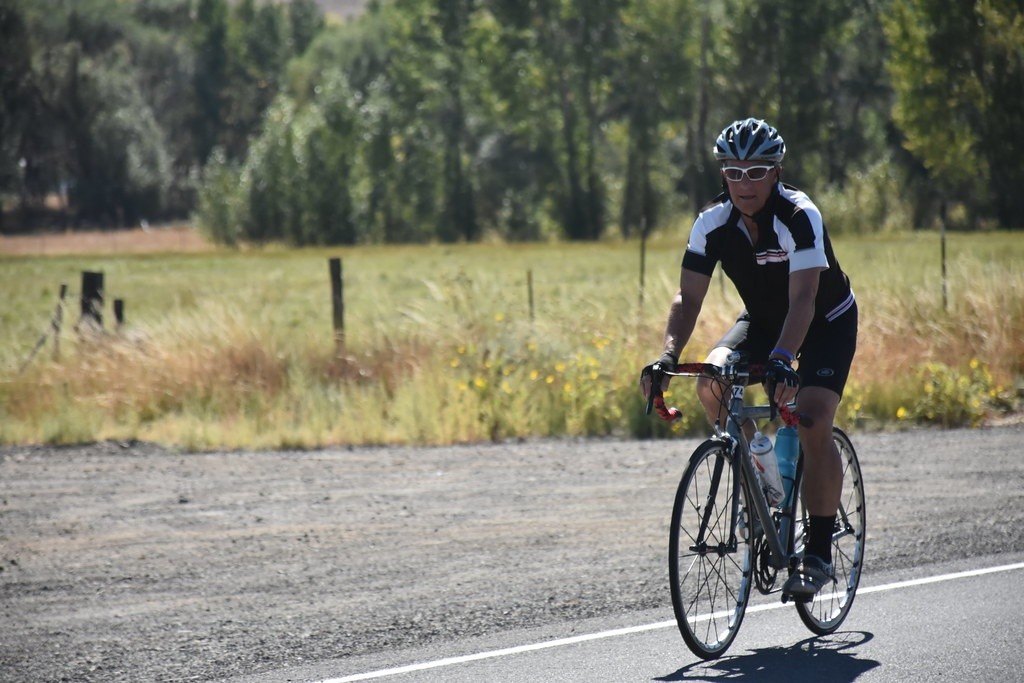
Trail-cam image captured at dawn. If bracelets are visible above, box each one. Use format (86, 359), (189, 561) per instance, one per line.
(771, 349), (794, 359)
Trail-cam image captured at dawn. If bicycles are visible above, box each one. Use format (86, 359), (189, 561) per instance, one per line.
(642, 359), (871, 662)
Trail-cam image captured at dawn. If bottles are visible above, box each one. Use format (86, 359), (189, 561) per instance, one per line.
(773, 426), (799, 508)
(750, 432), (785, 507)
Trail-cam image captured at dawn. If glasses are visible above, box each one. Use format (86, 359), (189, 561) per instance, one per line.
(720, 160), (775, 182)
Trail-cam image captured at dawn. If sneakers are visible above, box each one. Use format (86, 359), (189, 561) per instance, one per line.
(779, 554), (833, 594)
(739, 492), (770, 540)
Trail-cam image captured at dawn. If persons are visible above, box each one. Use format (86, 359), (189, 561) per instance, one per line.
(639, 117), (858, 595)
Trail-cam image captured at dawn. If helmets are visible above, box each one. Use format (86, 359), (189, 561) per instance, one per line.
(713, 118), (787, 162)
